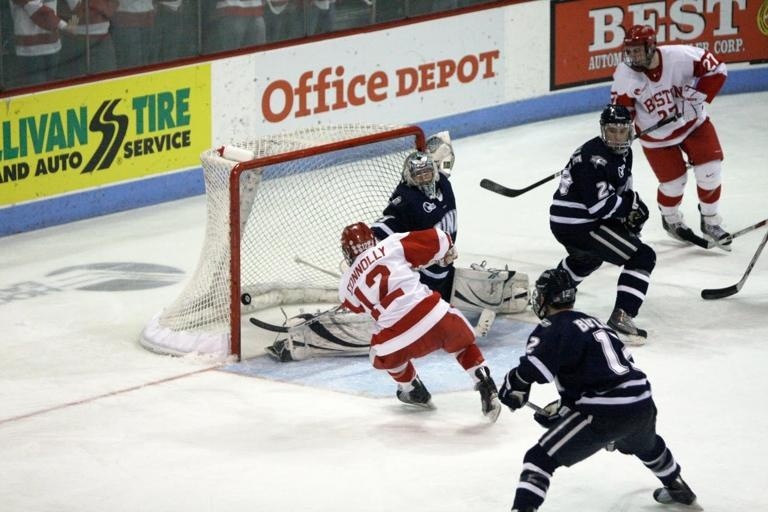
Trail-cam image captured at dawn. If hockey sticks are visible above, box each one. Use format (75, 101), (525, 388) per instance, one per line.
(498, 389), (617, 451)
(479, 110), (683, 198)
(700, 234), (767, 300)
(249, 248), (453, 333)
(672, 218), (767, 250)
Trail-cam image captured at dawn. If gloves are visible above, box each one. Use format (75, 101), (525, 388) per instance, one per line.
(682, 82), (708, 122)
(498, 367), (531, 412)
(626, 200), (650, 235)
(533, 399), (562, 430)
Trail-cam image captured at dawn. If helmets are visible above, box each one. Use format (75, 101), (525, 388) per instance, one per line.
(599, 104), (632, 153)
(532, 269), (577, 318)
(623, 24), (656, 69)
(402, 152), (440, 195)
(340, 221), (377, 268)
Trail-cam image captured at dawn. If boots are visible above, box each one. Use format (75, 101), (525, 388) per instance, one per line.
(658, 206), (695, 244)
(698, 204), (734, 252)
(653, 470), (698, 508)
(605, 308), (649, 347)
(475, 367), (501, 426)
(395, 374), (437, 412)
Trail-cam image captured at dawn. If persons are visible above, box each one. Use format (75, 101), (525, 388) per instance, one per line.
(10, 1), (373, 87)
(338, 223), (499, 416)
(550, 105), (656, 345)
(499, 268), (697, 512)
(265, 150), (531, 364)
(609, 24), (732, 246)
